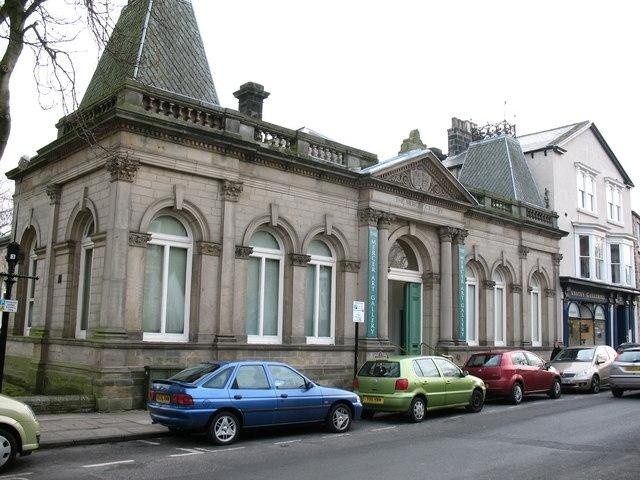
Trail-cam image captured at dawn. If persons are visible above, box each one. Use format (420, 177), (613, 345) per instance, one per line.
(550, 341), (564, 361)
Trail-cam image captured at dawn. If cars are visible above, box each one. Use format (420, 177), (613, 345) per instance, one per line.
(462, 349), (561, 405)
(353, 355), (486, 422)
(549, 344), (618, 393)
(147, 360), (363, 445)
(610, 342), (640, 396)
(0, 393), (43, 472)
